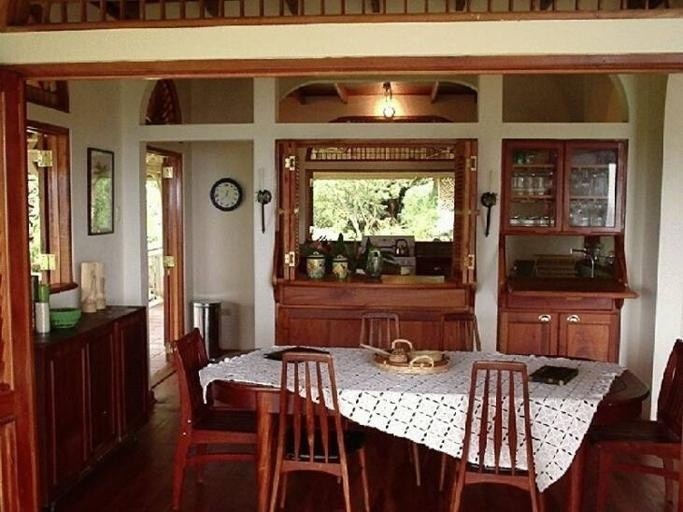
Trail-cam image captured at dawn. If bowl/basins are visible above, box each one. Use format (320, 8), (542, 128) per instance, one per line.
(49, 308), (82, 329)
(508, 216), (549, 226)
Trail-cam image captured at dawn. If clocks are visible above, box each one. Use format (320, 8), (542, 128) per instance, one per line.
(211, 179), (242, 213)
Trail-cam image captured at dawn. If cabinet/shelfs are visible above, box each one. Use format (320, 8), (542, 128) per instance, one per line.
(502, 138), (640, 297)
(498, 293), (621, 364)
(272, 275), (476, 353)
(32, 304), (149, 501)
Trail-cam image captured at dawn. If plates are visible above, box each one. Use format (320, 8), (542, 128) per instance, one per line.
(371, 349), (451, 375)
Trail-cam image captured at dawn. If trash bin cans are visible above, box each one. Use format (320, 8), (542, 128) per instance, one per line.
(189, 299), (222, 360)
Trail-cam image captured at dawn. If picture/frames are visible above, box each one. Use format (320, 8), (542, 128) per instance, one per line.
(86, 147), (116, 235)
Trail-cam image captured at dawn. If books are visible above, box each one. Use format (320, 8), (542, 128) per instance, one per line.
(264, 346), (330, 364)
(529, 365), (579, 387)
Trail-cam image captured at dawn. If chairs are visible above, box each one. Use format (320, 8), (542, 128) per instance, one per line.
(586, 337), (683, 512)
(438, 312), (481, 352)
(440, 361), (541, 511)
(266, 351), (370, 512)
(168, 330), (278, 512)
(336, 312), (422, 486)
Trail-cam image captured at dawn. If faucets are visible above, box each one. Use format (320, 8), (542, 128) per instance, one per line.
(570, 245), (615, 278)
